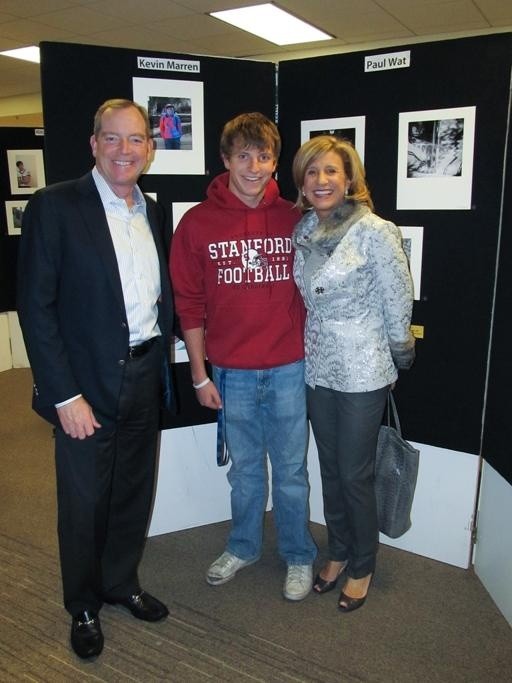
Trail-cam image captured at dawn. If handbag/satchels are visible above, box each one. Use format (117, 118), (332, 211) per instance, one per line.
(373, 425), (420, 539)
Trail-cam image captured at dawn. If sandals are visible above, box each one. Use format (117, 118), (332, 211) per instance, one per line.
(337, 567), (375, 614)
(312, 558), (350, 594)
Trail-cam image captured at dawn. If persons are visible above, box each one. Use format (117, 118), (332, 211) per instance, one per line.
(13, 98), (176, 662)
(168, 111), (321, 601)
(292, 136), (417, 613)
(159, 103), (184, 149)
(16, 160), (31, 189)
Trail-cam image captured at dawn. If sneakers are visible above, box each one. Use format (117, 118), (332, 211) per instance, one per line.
(206, 550), (262, 586)
(283, 565), (313, 601)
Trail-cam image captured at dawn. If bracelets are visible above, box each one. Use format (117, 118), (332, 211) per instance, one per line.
(193, 376), (211, 389)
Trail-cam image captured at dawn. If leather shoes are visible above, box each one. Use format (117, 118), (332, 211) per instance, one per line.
(115, 588), (170, 622)
(71, 613), (105, 662)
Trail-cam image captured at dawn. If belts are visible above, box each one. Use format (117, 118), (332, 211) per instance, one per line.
(128, 335), (166, 360)
